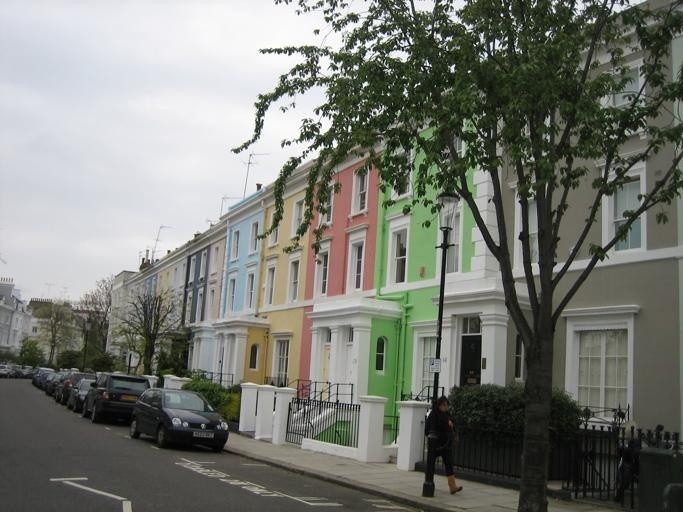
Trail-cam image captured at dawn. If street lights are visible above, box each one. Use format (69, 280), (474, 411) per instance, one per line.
(425, 185), (460, 481)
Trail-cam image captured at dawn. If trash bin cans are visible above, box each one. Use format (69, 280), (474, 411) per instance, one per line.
(639, 447), (683, 512)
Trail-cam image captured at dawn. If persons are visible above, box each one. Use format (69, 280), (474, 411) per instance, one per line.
(424, 395), (463, 495)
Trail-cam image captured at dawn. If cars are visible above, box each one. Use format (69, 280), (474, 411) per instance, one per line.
(0, 363), (151, 422)
(129, 387), (228, 452)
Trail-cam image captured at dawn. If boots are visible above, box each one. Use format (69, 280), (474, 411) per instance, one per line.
(447, 475), (462, 494)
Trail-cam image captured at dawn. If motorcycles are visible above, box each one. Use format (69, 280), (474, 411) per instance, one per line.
(613, 433), (675, 502)
